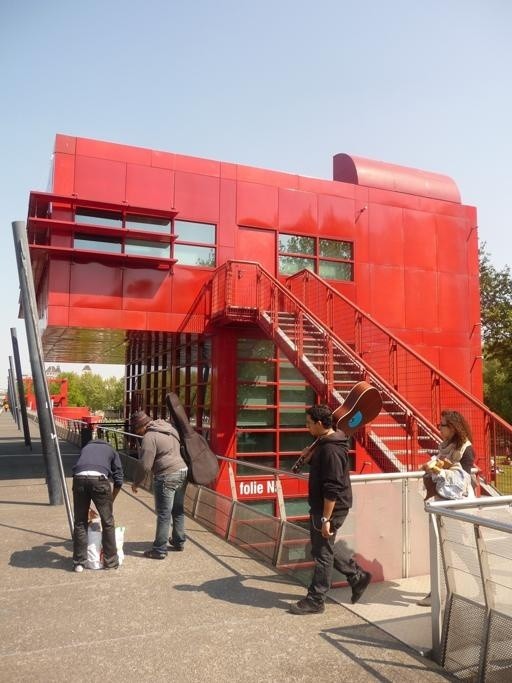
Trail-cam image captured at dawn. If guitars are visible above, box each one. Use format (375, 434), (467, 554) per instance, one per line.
(291, 380), (382, 474)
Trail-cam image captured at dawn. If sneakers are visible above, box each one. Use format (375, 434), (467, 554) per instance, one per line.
(75, 565), (84, 572)
(351, 571), (372, 603)
(418, 591), (433, 606)
(290, 599), (326, 613)
(144, 551), (166, 559)
(169, 536), (184, 551)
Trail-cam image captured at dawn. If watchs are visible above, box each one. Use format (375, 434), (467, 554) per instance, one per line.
(321, 516), (331, 523)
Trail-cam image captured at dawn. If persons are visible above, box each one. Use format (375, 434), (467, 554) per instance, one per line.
(417, 410), (475, 606)
(71, 439), (124, 573)
(129, 411), (190, 559)
(289, 404), (371, 615)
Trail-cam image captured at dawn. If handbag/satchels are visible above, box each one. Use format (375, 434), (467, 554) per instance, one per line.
(422, 473), (436, 500)
(87, 518), (125, 569)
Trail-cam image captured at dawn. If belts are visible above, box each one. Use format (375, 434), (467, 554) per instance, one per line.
(75, 475), (105, 480)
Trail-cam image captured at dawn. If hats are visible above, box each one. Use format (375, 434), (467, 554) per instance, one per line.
(130, 410), (154, 429)
